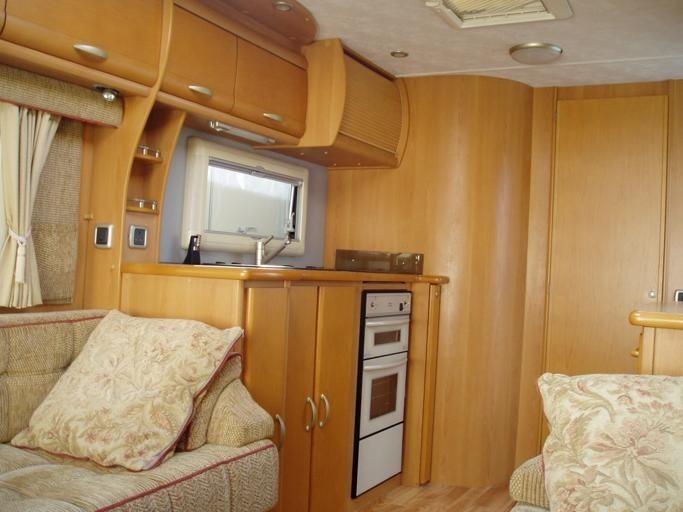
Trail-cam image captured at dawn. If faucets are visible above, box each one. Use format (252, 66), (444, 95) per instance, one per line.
(238, 225), (259, 234)
(262, 236), (292, 264)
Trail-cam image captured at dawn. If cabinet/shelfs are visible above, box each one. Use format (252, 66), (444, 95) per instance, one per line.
(119, 262), (362, 512)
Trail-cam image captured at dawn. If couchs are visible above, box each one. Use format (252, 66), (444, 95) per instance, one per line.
(0, 307), (278, 512)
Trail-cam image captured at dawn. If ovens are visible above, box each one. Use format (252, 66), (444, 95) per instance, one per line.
(352, 289), (414, 498)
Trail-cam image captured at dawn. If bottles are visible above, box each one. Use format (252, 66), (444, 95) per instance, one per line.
(182, 235), (202, 265)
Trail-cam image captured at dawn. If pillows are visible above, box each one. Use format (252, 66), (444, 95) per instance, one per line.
(8, 308), (243, 471)
(534, 367), (683, 511)
